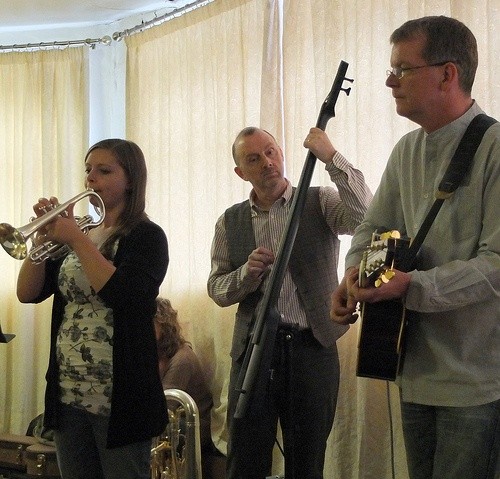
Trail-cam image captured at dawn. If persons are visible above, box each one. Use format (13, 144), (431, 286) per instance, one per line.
(208, 127), (374, 479)
(17, 139), (170, 479)
(330, 15), (500, 479)
(155, 297), (214, 479)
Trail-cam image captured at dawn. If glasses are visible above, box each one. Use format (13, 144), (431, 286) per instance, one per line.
(385, 60), (456, 80)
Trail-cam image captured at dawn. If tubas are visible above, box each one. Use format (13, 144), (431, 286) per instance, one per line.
(150, 388), (202, 479)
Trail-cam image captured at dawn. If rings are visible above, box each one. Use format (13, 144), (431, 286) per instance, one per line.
(307, 138), (311, 142)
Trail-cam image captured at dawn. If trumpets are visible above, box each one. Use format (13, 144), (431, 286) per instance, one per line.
(0, 186), (106, 266)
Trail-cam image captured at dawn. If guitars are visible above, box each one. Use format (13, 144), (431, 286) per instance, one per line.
(354, 226), (411, 383)
